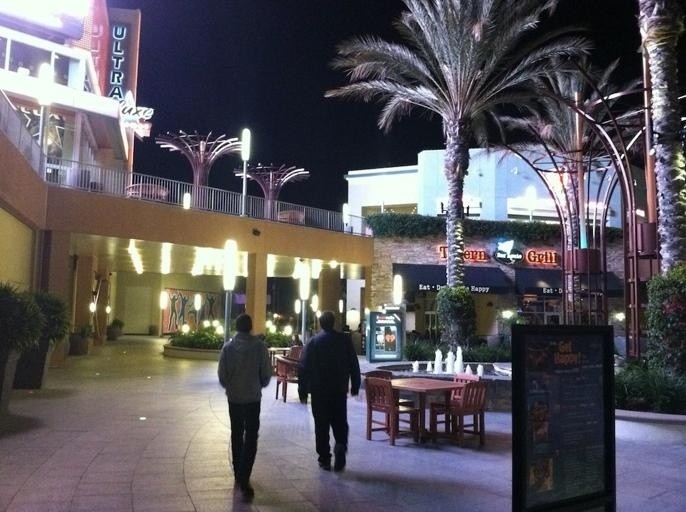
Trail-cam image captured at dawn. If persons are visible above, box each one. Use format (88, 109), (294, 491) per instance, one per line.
(357, 318), (367, 352)
(217, 312), (274, 499)
(295, 311), (362, 473)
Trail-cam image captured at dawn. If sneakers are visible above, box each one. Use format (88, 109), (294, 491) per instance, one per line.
(234, 477), (253, 498)
(333, 461), (346, 471)
(318, 457), (332, 472)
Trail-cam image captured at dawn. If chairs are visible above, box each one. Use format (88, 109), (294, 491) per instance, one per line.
(275, 346), (303, 402)
(364, 377), (420, 446)
(431, 383), (488, 448)
(431, 375), (481, 437)
(365, 371), (416, 434)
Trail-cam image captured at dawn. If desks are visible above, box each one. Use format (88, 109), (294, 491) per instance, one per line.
(387, 378), (467, 444)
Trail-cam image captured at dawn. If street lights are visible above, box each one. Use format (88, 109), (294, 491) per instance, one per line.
(393, 274), (402, 307)
(299, 263), (311, 346)
(221, 238), (238, 345)
(239, 129), (252, 218)
